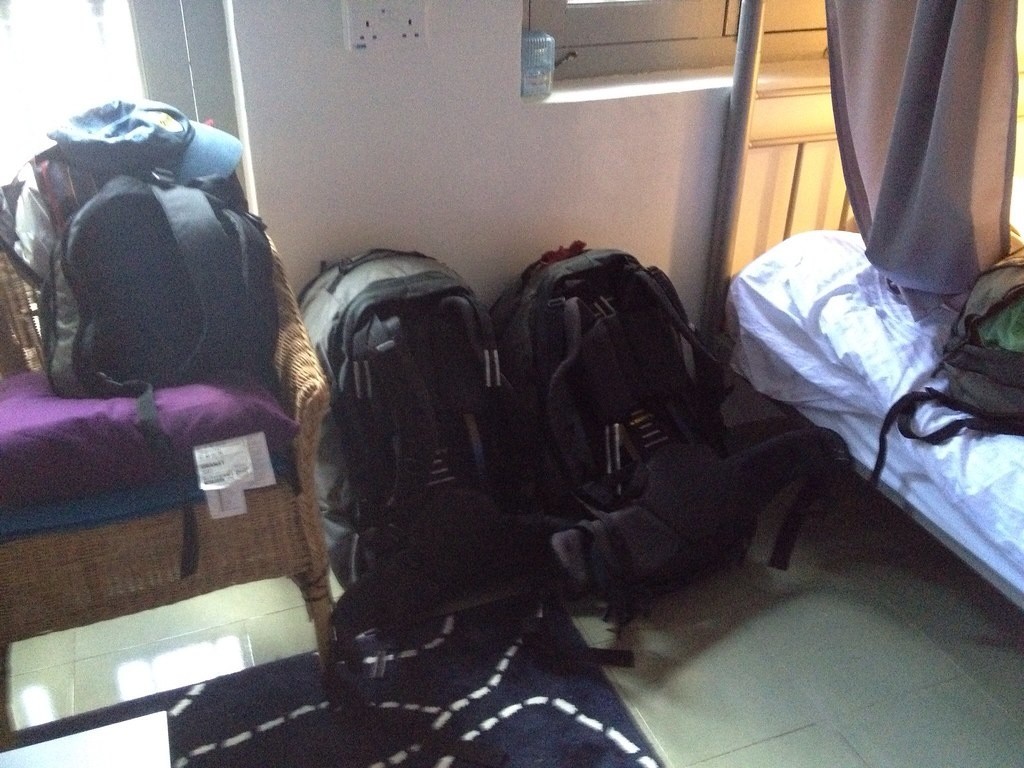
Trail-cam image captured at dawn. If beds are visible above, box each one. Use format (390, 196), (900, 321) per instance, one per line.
(719, 231), (1024, 610)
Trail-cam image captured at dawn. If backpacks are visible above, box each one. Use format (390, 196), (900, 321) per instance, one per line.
(496, 240), (787, 620)
(859, 251), (1024, 507)
(0, 102), (279, 400)
(296, 248), (524, 635)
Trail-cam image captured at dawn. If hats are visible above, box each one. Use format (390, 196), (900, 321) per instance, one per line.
(45, 98), (244, 188)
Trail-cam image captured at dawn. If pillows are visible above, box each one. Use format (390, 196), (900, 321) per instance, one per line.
(1, 379), (303, 494)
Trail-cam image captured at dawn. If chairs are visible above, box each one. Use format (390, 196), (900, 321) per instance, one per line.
(2, 181), (337, 741)
